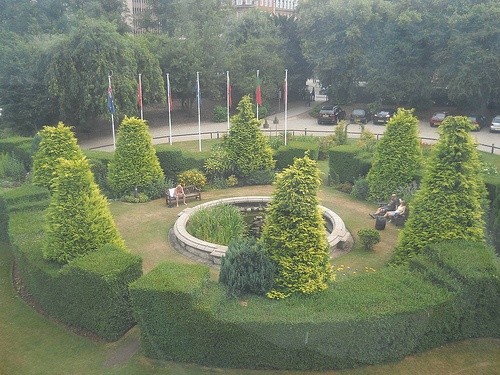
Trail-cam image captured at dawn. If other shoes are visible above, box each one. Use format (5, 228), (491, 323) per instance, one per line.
(184, 203), (187, 205)
(369, 214), (375, 219)
(177, 205), (179, 207)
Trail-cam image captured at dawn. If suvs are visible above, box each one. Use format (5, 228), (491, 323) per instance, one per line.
(317, 105), (346, 125)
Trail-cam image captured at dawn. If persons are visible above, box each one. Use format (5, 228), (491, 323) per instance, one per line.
(369, 194), (400, 219)
(173, 185), (187, 207)
(385, 200), (406, 218)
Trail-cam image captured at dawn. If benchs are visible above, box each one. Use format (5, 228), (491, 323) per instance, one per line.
(165, 186), (201, 207)
(379, 202), (408, 227)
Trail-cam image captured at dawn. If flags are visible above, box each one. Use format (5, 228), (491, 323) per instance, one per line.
(256, 78), (262, 106)
(138, 79), (142, 106)
(107, 81), (115, 116)
(228, 81), (232, 108)
(195, 79), (202, 105)
(284, 78), (287, 97)
(166, 78), (172, 112)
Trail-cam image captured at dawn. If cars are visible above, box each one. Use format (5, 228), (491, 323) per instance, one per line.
(429, 111), (456, 127)
(373, 108), (398, 125)
(319, 88), (326, 95)
(349, 108), (372, 124)
(489, 114), (500, 133)
(465, 115), (487, 131)
